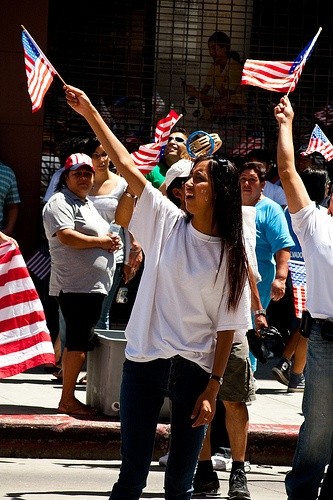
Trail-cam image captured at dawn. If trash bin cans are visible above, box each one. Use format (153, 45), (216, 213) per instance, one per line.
(87, 329), (129, 419)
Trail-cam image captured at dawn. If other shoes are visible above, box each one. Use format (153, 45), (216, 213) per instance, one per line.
(246, 326), (284, 363)
(159, 451), (170, 467)
(272, 356), (291, 386)
(209, 454), (234, 470)
(287, 373), (306, 391)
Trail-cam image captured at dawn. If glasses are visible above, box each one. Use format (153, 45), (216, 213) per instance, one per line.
(168, 137), (186, 143)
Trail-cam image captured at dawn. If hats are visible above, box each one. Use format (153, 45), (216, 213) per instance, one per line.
(65, 153), (95, 172)
(165, 159), (194, 190)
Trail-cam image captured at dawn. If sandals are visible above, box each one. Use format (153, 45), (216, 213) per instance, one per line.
(57, 400), (98, 416)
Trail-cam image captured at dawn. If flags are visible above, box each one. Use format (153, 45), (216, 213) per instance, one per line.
(0, 242), (56, 382)
(115, 109), (183, 176)
(99, 98), (114, 126)
(227, 136), (265, 156)
(26, 245), (52, 280)
(312, 104), (333, 126)
(238, 29), (321, 93)
(300, 124), (333, 161)
(140, 90), (164, 115)
(21, 29), (58, 113)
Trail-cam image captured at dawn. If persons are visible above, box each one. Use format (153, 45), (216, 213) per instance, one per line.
(0, 231), (19, 246)
(270, 94), (333, 500)
(61, 82), (253, 500)
(37, 82), (333, 500)
(0, 161), (22, 236)
(181, 33), (251, 123)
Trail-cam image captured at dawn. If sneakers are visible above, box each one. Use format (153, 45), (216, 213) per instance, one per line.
(228, 464), (252, 499)
(193, 472), (221, 495)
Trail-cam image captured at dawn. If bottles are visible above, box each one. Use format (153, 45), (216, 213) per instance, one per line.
(108, 218), (122, 239)
